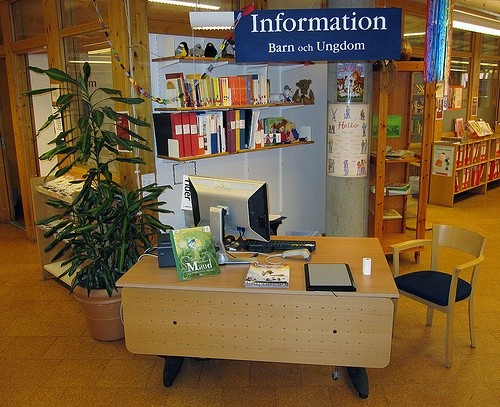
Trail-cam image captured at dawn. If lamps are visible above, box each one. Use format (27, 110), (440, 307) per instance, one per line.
(189, 10), (234, 30)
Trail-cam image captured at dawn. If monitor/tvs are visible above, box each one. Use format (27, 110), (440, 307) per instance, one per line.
(187, 176), (270, 266)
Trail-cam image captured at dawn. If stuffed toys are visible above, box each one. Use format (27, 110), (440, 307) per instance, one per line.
(270, 79), (315, 144)
(176, 32), (236, 57)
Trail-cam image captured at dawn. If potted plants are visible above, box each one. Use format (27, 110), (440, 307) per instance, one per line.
(20, 59), (176, 340)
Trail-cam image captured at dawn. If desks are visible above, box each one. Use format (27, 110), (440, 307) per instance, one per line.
(114, 235), (400, 398)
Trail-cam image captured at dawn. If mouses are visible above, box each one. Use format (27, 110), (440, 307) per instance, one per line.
(225, 235), (235, 245)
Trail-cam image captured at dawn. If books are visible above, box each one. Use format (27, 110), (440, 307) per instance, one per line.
(244, 262), (290, 288)
(170, 226), (220, 281)
(153, 73), (288, 157)
(369, 118), (500, 218)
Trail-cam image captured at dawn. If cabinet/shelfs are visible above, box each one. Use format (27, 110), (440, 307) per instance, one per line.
(408, 68), (500, 209)
(151, 55), (316, 161)
(29, 175), (111, 288)
(369, 60), (435, 263)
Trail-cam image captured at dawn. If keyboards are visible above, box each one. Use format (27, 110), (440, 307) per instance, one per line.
(244, 239), (315, 251)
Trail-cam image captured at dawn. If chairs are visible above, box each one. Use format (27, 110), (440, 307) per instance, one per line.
(387, 222), (490, 369)
